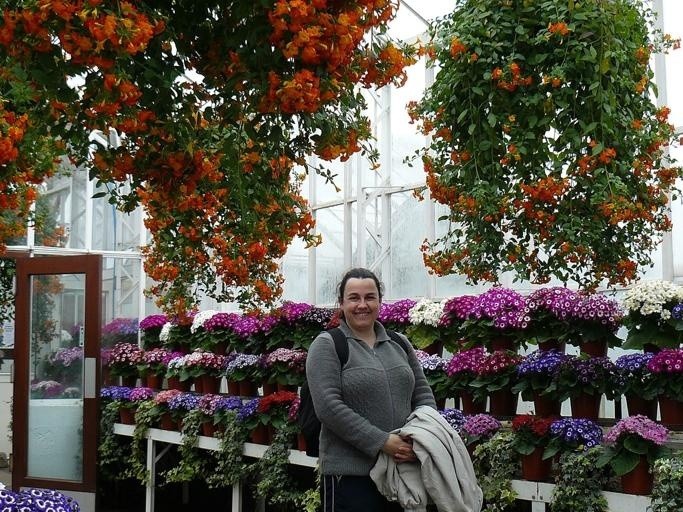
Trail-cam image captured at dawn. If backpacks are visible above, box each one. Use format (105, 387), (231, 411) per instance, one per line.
(296, 327), (409, 458)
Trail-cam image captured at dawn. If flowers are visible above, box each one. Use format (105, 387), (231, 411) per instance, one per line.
(31, 321), (84, 399)
(96, 279), (683, 512)
(0, 488), (80, 512)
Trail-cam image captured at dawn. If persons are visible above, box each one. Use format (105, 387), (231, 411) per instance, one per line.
(305, 268), (437, 512)
